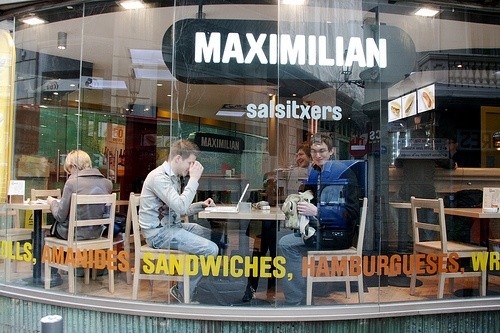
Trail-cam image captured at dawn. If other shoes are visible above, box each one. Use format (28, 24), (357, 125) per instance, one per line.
(41, 272), (64, 287)
(170, 285), (200, 305)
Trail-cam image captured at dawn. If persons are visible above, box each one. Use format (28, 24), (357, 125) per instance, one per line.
(396, 128), (438, 275)
(242, 172), (280, 302)
(442, 139), (464, 168)
(279, 133), (359, 307)
(46, 150), (113, 288)
(296, 141), (313, 192)
(139, 139), (218, 304)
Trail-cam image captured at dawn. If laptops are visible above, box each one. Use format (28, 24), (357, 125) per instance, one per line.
(205, 184), (249, 212)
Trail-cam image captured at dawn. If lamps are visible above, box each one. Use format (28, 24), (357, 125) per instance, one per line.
(58, 32), (67, 49)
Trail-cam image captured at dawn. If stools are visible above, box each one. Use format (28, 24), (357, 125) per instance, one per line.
(390, 201), (420, 256)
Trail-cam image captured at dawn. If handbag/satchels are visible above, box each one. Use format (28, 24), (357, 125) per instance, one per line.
(281, 189), (314, 230)
(114, 211), (133, 235)
(195, 272), (249, 306)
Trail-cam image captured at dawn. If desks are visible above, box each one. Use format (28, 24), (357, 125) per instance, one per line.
(5, 200), (129, 288)
(432, 208), (500, 297)
(198, 204), (285, 306)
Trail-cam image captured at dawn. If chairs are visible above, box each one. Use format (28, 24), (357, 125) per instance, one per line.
(0, 189), (190, 304)
(409, 196), (487, 300)
(306, 198), (368, 306)
